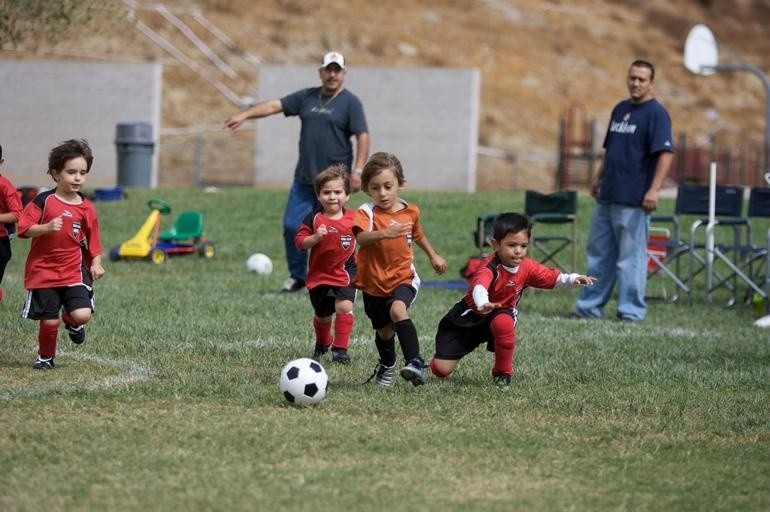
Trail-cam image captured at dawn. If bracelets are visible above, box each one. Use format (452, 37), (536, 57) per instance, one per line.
(353, 168), (363, 174)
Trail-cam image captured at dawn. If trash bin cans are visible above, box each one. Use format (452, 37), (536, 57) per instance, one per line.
(115, 122), (154, 187)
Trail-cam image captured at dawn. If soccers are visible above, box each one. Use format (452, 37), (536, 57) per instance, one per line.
(247, 254), (274, 274)
(281, 359), (329, 405)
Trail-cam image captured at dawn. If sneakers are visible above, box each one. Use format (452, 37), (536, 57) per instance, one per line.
(492, 373), (513, 394)
(374, 355), (399, 389)
(33, 355), (58, 371)
(399, 357), (427, 387)
(314, 340), (334, 362)
(331, 349), (351, 365)
(281, 276), (305, 294)
(61, 306), (85, 345)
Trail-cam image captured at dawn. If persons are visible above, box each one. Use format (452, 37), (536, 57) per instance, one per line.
(568, 60), (675, 321)
(294, 164), (357, 363)
(428, 212), (598, 391)
(16, 138), (105, 369)
(351, 151), (448, 387)
(222, 52), (370, 293)
(0, 145), (23, 301)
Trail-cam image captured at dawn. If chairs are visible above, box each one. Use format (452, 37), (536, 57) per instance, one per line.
(472, 190), (577, 273)
(688, 185), (770, 307)
(646, 186), (744, 306)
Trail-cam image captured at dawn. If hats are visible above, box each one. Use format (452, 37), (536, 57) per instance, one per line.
(320, 50), (346, 72)
